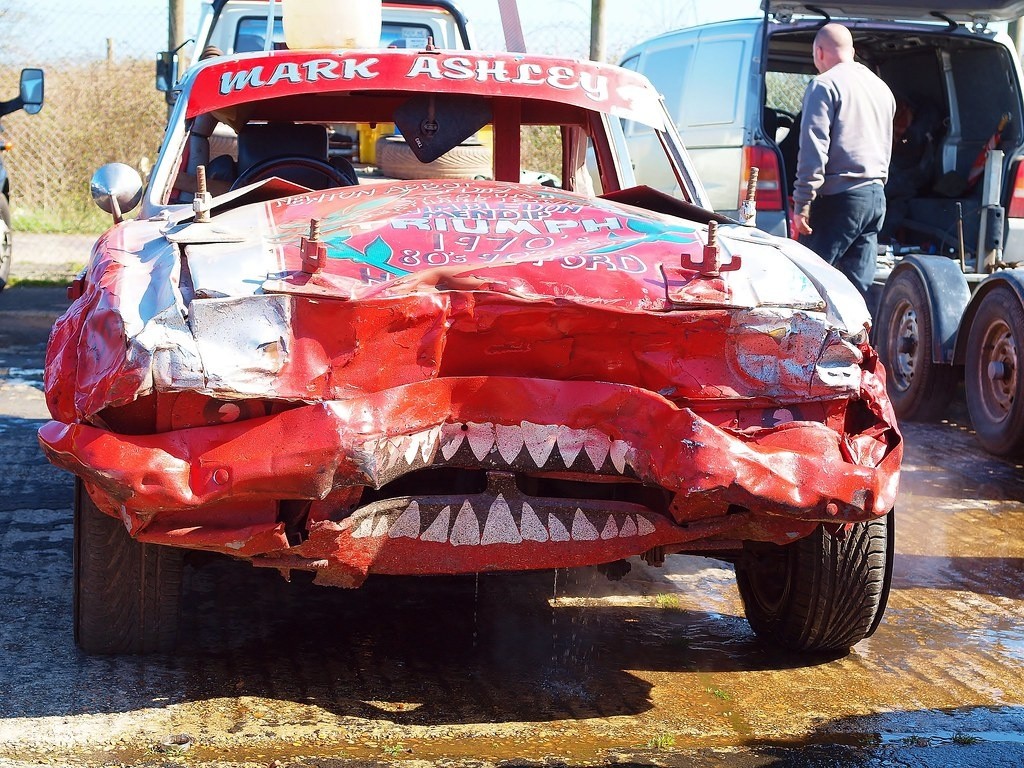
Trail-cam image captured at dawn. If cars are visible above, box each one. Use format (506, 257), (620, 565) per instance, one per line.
(39, 46), (902, 667)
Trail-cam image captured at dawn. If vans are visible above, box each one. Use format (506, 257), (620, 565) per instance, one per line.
(586, 0), (1023, 301)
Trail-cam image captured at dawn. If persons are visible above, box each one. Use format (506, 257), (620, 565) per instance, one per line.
(793, 23), (896, 292)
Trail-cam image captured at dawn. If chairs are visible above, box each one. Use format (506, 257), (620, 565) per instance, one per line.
(237, 123), (329, 199)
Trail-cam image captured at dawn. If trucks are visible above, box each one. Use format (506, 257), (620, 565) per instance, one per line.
(1, 67), (47, 287)
(157, 0), (561, 207)
